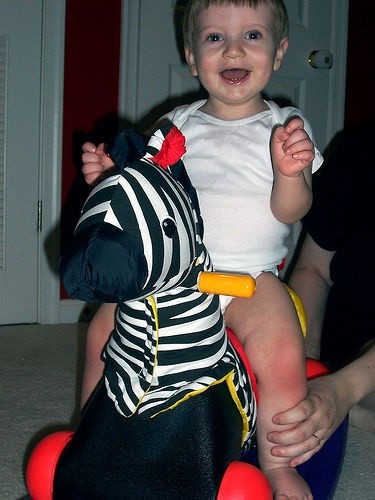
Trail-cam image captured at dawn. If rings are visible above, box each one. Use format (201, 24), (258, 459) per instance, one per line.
(313, 434), (321, 442)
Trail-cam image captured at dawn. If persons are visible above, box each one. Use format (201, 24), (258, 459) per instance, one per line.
(267, 124), (375, 469)
(78, 0), (324, 500)
